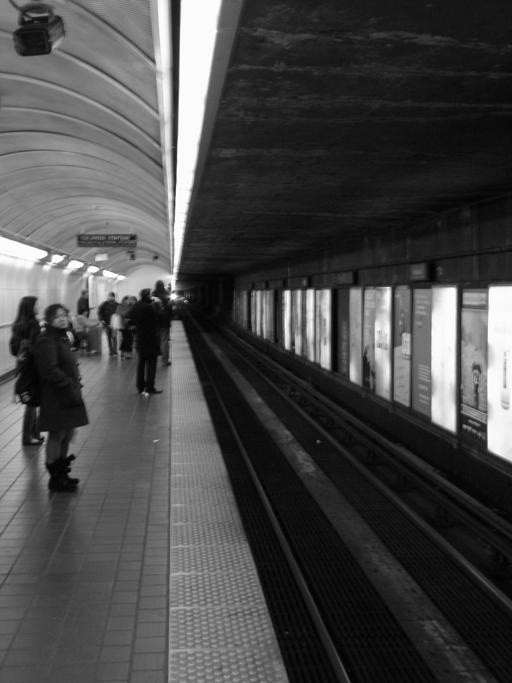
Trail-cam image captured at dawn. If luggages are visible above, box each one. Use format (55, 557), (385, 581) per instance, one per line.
(88, 326), (102, 354)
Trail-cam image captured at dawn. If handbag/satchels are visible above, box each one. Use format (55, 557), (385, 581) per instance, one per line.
(111, 314), (121, 329)
(17, 339), (32, 361)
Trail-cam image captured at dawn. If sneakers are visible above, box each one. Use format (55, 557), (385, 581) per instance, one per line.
(22, 438), (44, 445)
(145, 389), (161, 394)
(138, 388), (144, 394)
(163, 362), (171, 367)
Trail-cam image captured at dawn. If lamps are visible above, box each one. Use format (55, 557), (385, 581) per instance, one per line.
(6, 0), (66, 57)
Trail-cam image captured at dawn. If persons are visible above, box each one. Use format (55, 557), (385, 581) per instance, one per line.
(70, 280), (171, 394)
(12, 295), (45, 446)
(33, 303), (89, 493)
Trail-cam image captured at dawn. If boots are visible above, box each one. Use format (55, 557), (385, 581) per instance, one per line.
(47, 453), (78, 490)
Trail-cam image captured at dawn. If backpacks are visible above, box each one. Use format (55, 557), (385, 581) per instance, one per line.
(15, 357), (39, 406)
